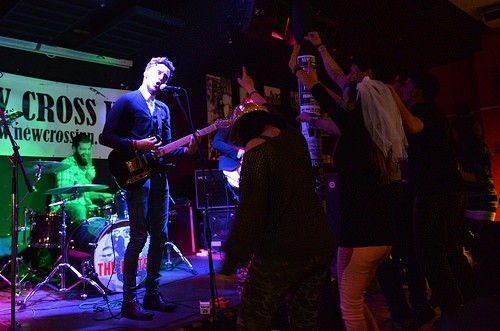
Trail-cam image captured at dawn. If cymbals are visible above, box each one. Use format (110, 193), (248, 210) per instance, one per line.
(44, 185), (108, 195)
(23, 161), (70, 174)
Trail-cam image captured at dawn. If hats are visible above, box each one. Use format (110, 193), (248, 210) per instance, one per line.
(230, 97), (270, 147)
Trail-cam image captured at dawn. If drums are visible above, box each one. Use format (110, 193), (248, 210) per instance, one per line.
(29, 211), (70, 248)
(71, 216), (150, 293)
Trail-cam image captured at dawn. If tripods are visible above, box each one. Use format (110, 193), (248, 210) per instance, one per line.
(19, 183), (107, 311)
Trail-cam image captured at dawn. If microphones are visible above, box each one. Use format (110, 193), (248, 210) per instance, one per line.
(160, 83), (183, 92)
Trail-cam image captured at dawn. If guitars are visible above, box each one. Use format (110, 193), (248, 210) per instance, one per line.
(108, 118), (231, 191)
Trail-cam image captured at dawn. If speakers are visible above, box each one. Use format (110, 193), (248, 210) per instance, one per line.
(194, 169), (240, 209)
(167, 200), (196, 257)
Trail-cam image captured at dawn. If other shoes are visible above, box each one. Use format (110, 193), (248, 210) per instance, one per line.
(392, 319), (415, 331)
(122, 300), (155, 319)
(142, 291), (177, 311)
(411, 307), (434, 327)
